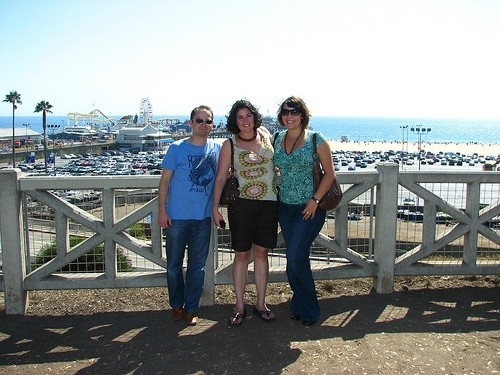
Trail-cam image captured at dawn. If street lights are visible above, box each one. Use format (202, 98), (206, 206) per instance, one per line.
(22, 123), (30, 158)
(47, 123), (61, 177)
(411, 127), (431, 205)
(399, 124), (409, 170)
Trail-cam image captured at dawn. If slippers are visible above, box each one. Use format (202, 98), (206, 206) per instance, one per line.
(228, 308), (247, 327)
(253, 305), (276, 321)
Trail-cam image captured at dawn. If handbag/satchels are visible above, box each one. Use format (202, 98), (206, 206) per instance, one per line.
(311, 132), (343, 212)
(219, 175), (240, 205)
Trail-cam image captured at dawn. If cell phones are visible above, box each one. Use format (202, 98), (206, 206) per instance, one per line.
(220, 220), (226, 229)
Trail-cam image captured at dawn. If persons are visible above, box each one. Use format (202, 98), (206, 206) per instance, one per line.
(271, 96), (335, 325)
(213, 99), (279, 327)
(158, 105), (221, 326)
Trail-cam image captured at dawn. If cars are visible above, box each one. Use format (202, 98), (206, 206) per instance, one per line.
(331, 148), (500, 171)
(2, 135), (168, 205)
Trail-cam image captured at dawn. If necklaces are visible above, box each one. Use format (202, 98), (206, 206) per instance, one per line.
(238, 129), (257, 142)
(284, 128), (304, 156)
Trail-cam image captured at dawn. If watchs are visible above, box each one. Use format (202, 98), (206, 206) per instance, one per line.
(311, 196), (319, 204)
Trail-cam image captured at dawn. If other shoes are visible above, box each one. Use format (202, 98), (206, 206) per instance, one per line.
(173, 308), (184, 321)
(183, 311), (199, 326)
(291, 314), (301, 320)
(302, 320), (315, 326)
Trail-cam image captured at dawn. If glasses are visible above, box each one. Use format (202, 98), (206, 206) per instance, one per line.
(280, 108), (301, 116)
(195, 118), (213, 124)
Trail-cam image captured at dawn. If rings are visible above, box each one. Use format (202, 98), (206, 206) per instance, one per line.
(306, 211), (309, 214)
